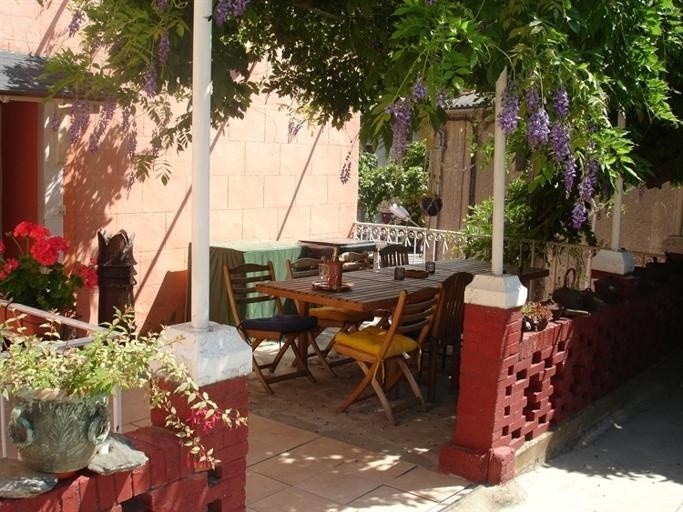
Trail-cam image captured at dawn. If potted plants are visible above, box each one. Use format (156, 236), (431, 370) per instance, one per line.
(521, 302), (553, 332)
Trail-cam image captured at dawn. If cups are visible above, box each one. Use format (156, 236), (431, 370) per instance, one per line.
(425, 262), (436, 274)
(392, 266), (405, 280)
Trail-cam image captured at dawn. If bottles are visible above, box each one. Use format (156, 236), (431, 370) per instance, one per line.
(328, 246), (342, 287)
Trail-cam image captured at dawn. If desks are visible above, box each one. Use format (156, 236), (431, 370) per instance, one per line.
(187, 241), (375, 326)
(257, 257), (550, 397)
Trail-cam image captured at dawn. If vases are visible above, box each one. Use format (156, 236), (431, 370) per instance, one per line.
(0, 300), (76, 341)
(3, 388), (110, 481)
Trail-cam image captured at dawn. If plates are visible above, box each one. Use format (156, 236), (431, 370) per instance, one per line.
(311, 280), (357, 292)
(403, 269), (427, 279)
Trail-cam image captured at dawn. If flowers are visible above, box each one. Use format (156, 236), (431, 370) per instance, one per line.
(0, 293), (250, 470)
(0, 219), (98, 316)
